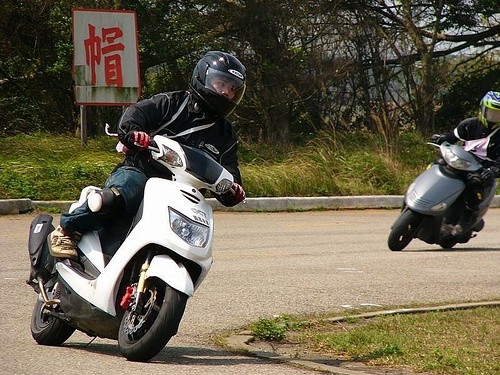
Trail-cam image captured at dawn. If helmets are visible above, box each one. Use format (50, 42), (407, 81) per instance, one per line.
(477, 90), (500, 130)
(189, 50), (247, 118)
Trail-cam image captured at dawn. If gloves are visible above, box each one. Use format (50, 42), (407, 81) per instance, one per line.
(230, 183), (246, 202)
(480, 166), (500, 183)
(432, 133), (451, 145)
(122, 131), (152, 151)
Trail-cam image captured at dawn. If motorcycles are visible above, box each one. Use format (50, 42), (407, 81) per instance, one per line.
(388, 133), (499, 251)
(26, 134), (247, 361)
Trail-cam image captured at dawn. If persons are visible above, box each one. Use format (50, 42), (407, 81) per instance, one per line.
(47, 51), (247, 261)
(432, 91), (500, 238)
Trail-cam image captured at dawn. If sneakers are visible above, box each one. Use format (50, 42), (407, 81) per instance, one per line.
(47, 224), (82, 260)
